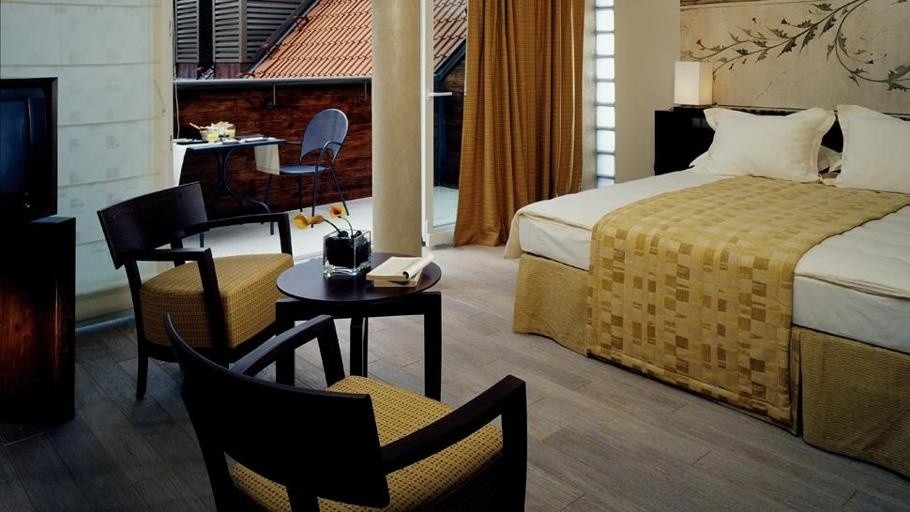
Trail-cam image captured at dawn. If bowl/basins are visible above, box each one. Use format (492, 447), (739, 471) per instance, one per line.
(199, 124), (237, 143)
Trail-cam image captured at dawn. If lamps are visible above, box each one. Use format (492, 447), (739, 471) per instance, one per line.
(674, 61), (713, 107)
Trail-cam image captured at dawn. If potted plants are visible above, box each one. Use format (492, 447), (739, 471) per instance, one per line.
(292, 203), (374, 280)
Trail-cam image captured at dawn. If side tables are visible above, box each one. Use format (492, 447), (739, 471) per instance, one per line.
(275, 252), (442, 402)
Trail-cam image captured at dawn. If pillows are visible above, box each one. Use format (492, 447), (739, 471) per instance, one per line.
(688, 103), (909, 195)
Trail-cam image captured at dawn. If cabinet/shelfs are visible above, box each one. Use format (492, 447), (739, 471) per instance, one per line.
(0, 218), (78, 429)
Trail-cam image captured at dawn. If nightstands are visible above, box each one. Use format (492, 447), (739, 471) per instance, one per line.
(654, 110), (714, 172)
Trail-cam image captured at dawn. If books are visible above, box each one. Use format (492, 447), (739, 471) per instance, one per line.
(365, 253), (435, 289)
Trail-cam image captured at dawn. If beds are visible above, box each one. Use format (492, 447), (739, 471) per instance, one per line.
(503, 106), (910, 480)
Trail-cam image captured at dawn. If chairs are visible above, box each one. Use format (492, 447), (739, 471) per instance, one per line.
(96, 180), (294, 401)
(162, 310), (527, 511)
(261, 108), (349, 228)
